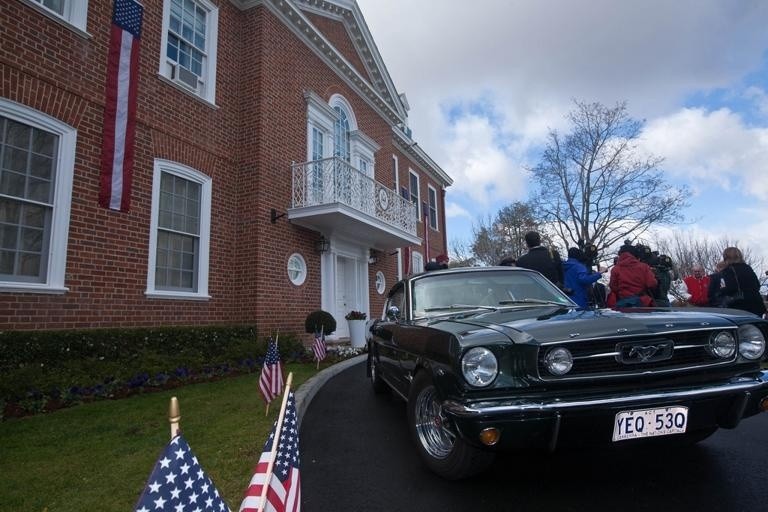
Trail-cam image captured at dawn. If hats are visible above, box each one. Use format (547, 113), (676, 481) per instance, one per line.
(436, 255), (449, 264)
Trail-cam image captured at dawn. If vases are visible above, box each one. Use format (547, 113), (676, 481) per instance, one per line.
(347, 320), (366, 348)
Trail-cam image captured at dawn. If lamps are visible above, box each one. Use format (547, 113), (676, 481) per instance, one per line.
(316, 236), (330, 255)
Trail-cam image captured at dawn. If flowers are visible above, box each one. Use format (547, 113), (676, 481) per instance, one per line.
(345, 310), (367, 320)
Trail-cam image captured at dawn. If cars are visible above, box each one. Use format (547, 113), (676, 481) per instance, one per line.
(365, 266), (766, 482)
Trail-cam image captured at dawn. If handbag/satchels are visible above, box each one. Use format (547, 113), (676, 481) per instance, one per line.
(718, 267), (745, 311)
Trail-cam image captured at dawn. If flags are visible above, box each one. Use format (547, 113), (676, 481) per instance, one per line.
(312, 328), (327, 362)
(258, 336), (285, 405)
(238, 390), (302, 512)
(136, 431), (234, 512)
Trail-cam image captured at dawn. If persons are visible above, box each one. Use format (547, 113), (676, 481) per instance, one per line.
(499, 230), (768, 319)
(424, 254), (449, 271)
